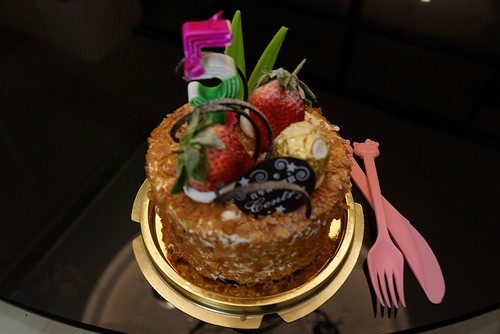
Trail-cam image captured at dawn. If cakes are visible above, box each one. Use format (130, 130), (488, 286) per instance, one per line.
(145, 10), (353, 299)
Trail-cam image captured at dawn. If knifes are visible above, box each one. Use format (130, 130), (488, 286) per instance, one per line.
(347, 155), (445, 304)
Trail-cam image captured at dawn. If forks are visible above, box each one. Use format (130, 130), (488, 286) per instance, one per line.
(353, 139), (406, 309)
